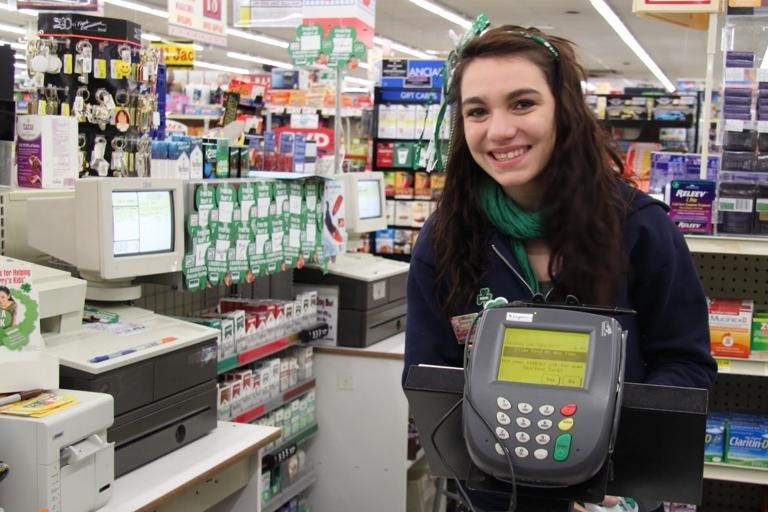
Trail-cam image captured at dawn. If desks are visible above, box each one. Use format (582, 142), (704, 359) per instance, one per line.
(81, 419), (282, 512)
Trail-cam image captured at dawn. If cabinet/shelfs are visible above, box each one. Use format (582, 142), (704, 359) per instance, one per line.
(214, 320), (329, 512)
(369, 74), (768, 511)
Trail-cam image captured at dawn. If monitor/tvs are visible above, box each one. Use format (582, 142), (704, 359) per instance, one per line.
(404, 362), (709, 506)
(25, 176), (186, 301)
(332, 172), (388, 249)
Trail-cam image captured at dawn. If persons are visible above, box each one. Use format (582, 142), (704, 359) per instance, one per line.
(400, 13), (720, 512)
(0, 286), (17, 328)
